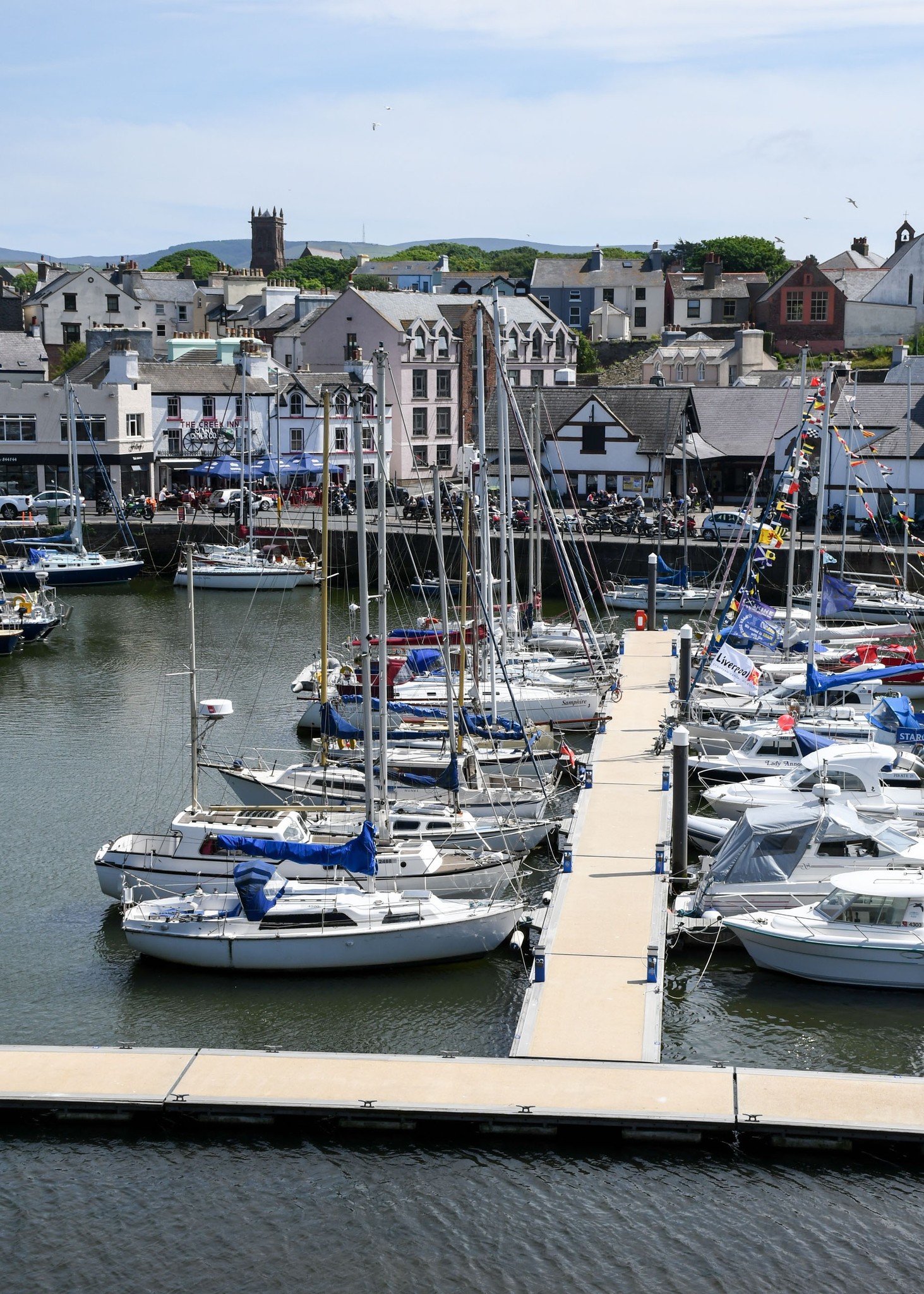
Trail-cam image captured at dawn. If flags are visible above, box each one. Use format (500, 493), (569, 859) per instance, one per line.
(709, 377), (924, 696)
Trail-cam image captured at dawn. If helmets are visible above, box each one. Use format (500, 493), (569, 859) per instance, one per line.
(243, 486), (247, 491)
(139, 490), (144, 494)
(338, 488), (344, 492)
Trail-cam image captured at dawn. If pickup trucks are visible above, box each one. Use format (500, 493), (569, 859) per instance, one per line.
(0, 484), (33, 521)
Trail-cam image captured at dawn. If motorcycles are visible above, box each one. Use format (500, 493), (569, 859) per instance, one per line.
(328, 494), (356, 516)
(221, 496), (259, 518)
(554, 503), (699, 541)
(117, 494), (154, 520)
(97, 490), (114, 516)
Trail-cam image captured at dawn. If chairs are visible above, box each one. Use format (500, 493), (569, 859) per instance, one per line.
(672, 502), (685, 516)
(651, 501), (659, 518)
(700, 498), (714, 513)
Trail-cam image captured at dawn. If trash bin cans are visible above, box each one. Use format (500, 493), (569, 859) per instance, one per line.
(547, 490), (556, 507)
(47, 506), (61, 525)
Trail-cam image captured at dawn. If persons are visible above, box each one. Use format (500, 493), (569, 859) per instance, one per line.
(139, 480), (699, 643)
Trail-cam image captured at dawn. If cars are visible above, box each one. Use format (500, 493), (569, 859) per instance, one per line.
(208, 488), (274, 513)
(699, 511), (774, 544)
(20, 489), (86, 516)
(23, 483), (76, 499)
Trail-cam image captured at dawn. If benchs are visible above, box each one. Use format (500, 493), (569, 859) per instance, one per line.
(619, 510), (631, 514)
(613, 511), (620, 516)
(591, 512), (603, 516)
(402, 501), (462, 523)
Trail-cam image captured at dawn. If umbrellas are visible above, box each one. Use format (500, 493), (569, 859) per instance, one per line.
(187, 453), (343, 489)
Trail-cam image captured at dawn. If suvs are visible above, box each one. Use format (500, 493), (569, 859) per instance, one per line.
(346, 477), (410, 509)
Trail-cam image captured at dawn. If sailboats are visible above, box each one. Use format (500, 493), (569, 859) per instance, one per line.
(666, 355), (924, 995)
(0, 264), (624, 978)
(602, 406), (735, 613)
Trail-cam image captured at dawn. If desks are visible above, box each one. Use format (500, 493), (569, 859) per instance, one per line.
(590, 502), (632, 519)
